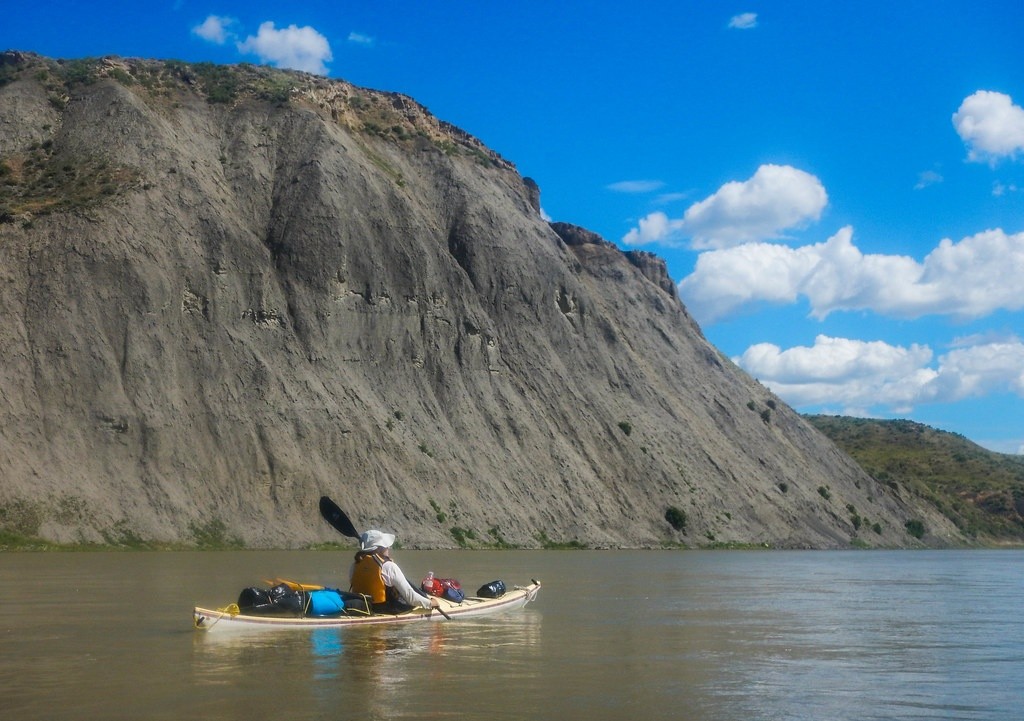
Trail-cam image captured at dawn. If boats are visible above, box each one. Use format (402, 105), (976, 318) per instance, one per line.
(193, 574), (541, 632)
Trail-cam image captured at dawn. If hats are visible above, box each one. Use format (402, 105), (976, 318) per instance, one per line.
(360, 530), (395, 552)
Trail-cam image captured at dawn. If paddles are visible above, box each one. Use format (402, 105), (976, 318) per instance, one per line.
(319, 496), (451, 620)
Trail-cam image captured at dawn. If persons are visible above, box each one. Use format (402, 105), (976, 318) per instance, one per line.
(350, 530), (439, 615)
